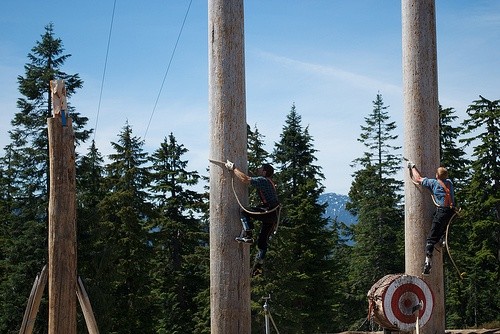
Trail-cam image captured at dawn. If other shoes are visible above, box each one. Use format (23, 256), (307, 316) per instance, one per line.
(235, 235), (254, 243)
(250, 263), (264, 278)
(422, 261), (432, 276)
(439, 234), (446, 247)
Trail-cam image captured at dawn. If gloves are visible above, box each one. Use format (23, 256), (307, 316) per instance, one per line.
(408, 161), (415, 169)
(225, 159), (235, 171)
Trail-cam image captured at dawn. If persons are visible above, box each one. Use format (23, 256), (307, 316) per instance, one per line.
(407, 159), (457, 277)
(224, 159), (281, 278)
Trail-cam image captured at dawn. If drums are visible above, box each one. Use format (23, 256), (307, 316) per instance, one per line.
(366, 273), (435, 331)
